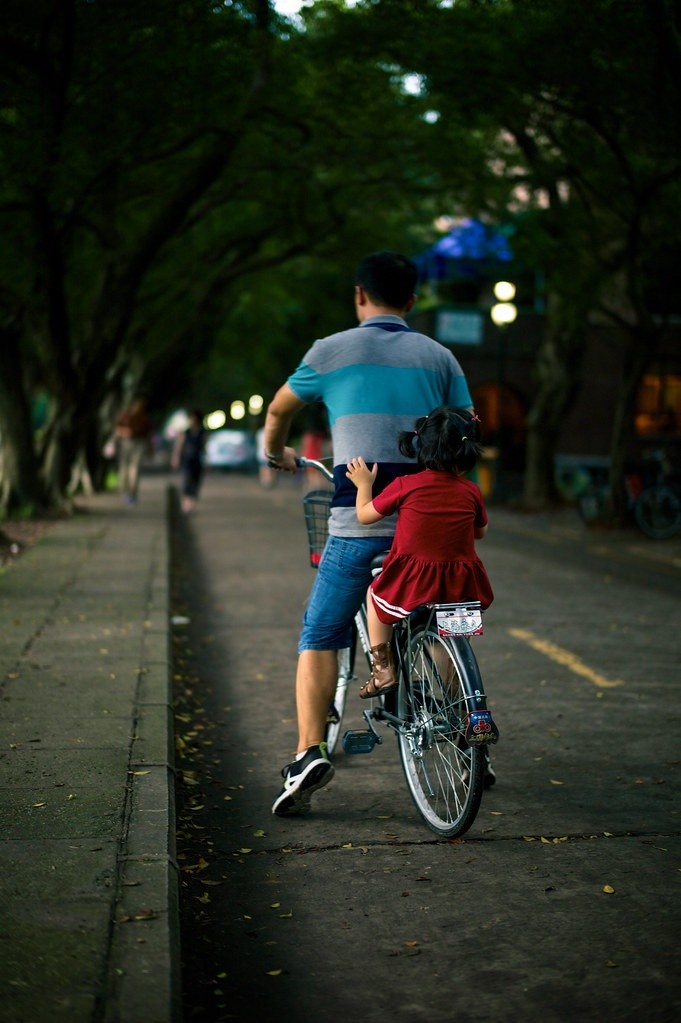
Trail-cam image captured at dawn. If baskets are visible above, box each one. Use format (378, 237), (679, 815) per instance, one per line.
(303, 491), (335, 568)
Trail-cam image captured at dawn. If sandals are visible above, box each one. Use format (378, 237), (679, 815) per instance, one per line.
(359, 642), (397, 699)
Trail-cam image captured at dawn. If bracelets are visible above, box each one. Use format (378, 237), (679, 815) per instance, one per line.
(265, 449), (284, 460)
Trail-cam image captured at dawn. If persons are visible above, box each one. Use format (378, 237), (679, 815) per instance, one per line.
(264, 255), (497, 815)
(256, 423), (323, 492)
(347, 407), (494, 699)
(172, 411), (206, 513)
(104, 400), (153, 505)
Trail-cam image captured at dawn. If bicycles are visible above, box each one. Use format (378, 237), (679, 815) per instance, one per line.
(266, 457), (501, 841)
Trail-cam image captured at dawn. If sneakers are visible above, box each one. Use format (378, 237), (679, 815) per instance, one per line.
(460, 754), (496, 790)
(272, 741), (335, 818)
